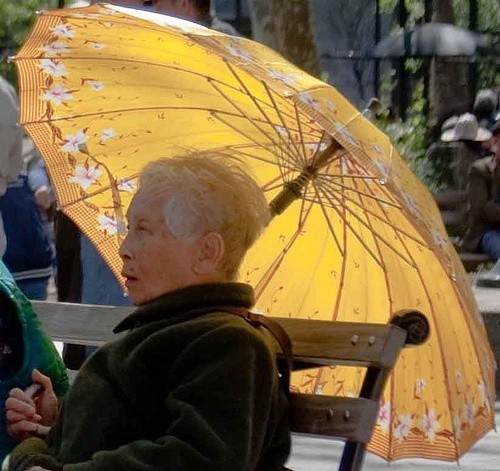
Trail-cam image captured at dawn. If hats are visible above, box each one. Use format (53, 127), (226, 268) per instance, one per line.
(441, 113), (492, 141)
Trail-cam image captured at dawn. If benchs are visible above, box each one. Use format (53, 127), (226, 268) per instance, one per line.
(432, 190), (498, 271)
(28, 300), (430, 470)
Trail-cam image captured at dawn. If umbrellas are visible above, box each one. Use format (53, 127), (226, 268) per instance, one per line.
(8, 2), (500, 467)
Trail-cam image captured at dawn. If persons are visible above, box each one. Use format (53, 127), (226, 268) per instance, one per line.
(0, 69), (86, 373)
(156, 0), (242, 37)
(361, 98), (383, 124)
(2, 146), (290, 471)
(419, 88), (500, 293)
